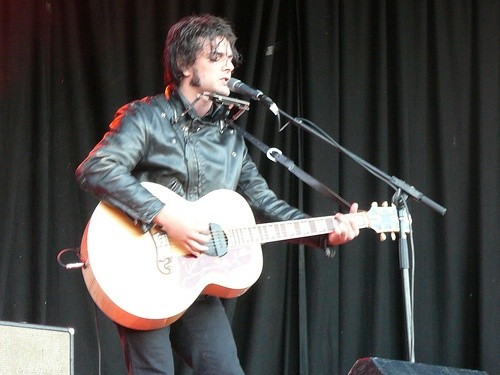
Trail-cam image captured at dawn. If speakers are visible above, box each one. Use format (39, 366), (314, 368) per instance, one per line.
(0, 319), (75, 375)
(349, 357), (487, 375)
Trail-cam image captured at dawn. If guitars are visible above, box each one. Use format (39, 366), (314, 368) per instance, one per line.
(79, 181), (413, 330)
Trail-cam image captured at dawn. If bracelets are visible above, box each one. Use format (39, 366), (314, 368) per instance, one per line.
(154, 211), (166, 231)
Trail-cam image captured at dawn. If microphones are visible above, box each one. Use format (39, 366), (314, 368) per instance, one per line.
(227, 78), (275, 108)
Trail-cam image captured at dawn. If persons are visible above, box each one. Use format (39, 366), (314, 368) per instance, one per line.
(74, 15), (360, 375)
